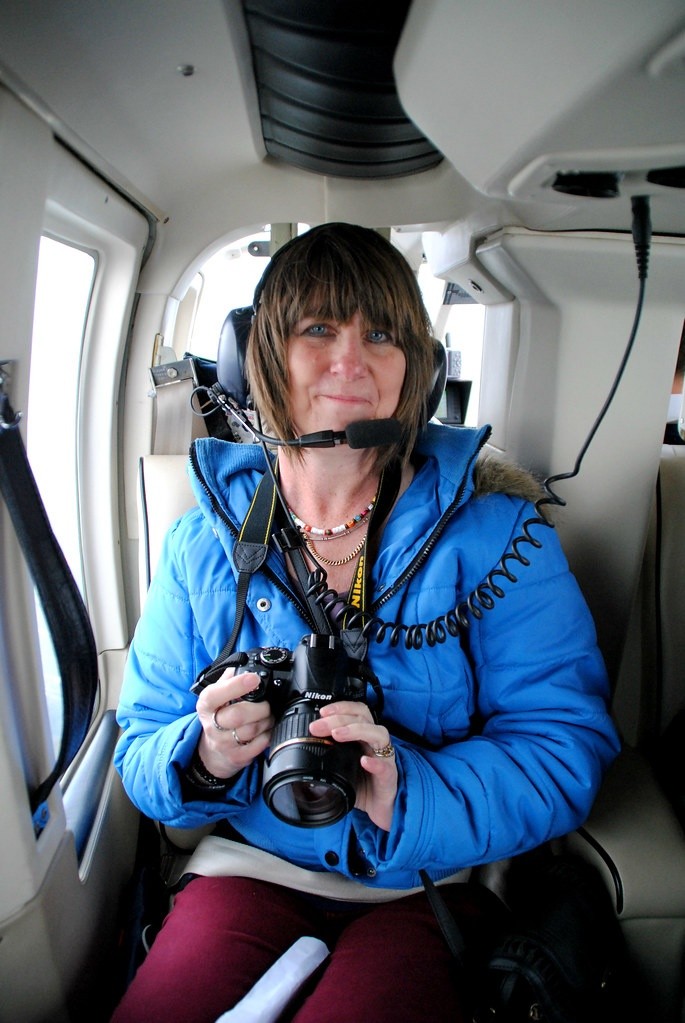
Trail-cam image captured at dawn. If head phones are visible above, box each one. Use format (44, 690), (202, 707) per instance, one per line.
(189, 221), (449, 422)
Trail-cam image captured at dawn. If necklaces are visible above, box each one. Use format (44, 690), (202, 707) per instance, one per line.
(287, 487), (378, 534)
(298, 512), (372, 540)
(302, 534), (367, 566)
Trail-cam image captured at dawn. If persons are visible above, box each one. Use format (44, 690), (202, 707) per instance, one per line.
(112, 222), (623, 1023)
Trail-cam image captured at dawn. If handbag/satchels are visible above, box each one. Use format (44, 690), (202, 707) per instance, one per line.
(419, 834), (660, 1023)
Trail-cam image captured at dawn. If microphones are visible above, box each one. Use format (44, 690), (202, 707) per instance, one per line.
(216, 396), (401, 449)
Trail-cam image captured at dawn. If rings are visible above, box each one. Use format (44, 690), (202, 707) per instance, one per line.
(232, 728), (245, 744)
(372, 741), (396, 758)
(213, 707), (230, 731)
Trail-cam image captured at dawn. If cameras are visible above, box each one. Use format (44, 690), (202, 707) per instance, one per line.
(226, 633), (370, 830)
